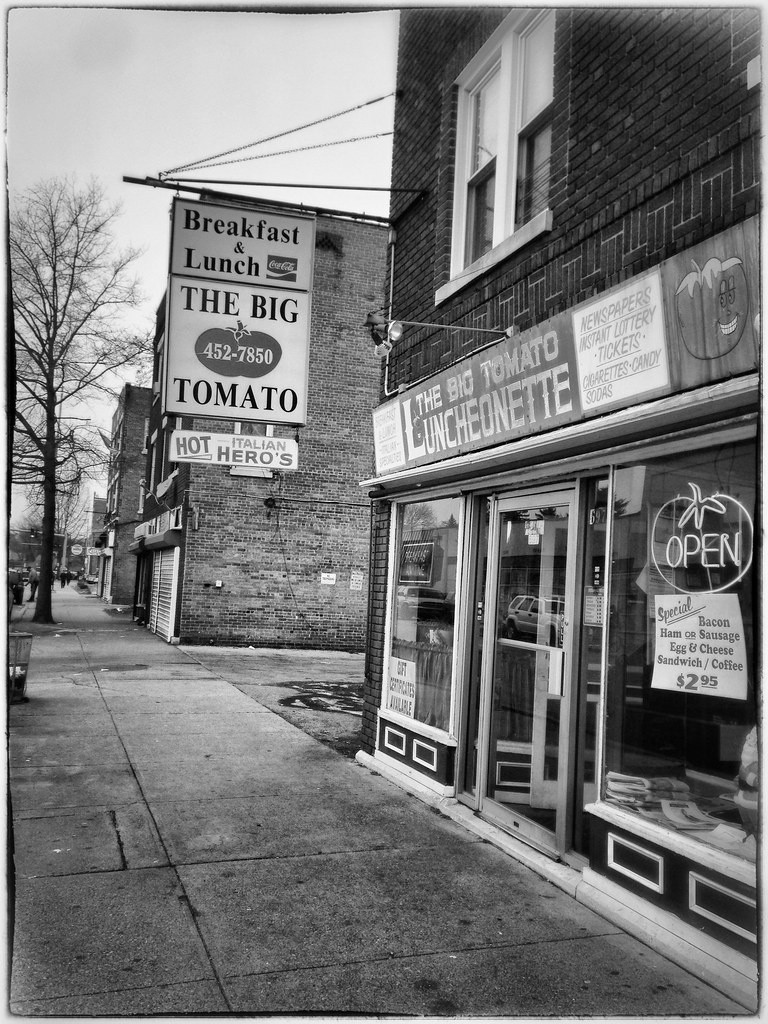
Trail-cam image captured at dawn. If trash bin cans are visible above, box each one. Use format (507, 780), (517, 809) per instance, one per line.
(9, 630), (33, 705)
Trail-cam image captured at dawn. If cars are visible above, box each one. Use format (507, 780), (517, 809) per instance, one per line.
(396, 586), (449, 617)
(6, 570), (25, 617)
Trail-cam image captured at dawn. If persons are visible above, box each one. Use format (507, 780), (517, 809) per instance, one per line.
(734, 723), (759, 832)
(60, 570), (73, 588)
(51, 570), (55, 590)
(25, 566), (40, 602)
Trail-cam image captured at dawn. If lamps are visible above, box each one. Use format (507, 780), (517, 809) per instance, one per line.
(139, 479), (165, 508)
(363, 313), (513, 358)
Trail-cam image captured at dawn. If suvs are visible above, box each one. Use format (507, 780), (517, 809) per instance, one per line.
(506, 594), (566, 644)
